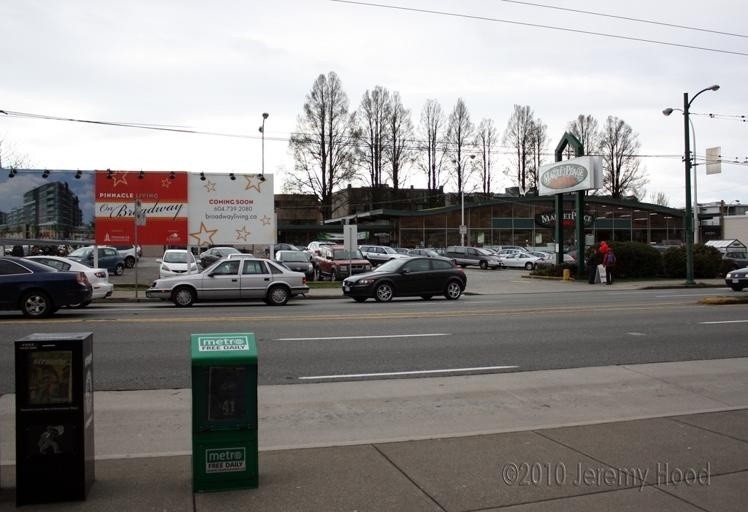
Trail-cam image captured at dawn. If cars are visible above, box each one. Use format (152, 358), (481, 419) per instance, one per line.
(155, 249), (202, 280)
(21, 256), (114, 310)
(0, 255), (93, 321)
(4, 241), (142, 276)
(146, 254), (310, 306)
(725, 266), (747, 291)
(342, 257), (467, 303)
(200, 247), (242, 269)
(274, 249), (314, 281)
(647, 239), (686, 255)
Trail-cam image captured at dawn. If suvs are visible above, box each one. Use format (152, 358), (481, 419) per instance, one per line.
(311, 245), (371, 283)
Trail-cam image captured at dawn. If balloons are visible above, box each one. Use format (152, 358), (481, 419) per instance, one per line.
(598, 241), (608, 253)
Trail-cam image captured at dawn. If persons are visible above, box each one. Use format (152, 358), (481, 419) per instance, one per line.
(586, 249), (601, 285)
(602, 248), (616, 286)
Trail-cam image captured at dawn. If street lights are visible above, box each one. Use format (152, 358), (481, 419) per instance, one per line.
(662, 108), (699, 245)
(683, 83), (720, 287)
(451, 154), (476, 246)
(258, 112), (269, 174)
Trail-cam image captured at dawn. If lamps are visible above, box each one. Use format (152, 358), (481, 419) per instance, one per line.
(8, 168), (266, 184)
